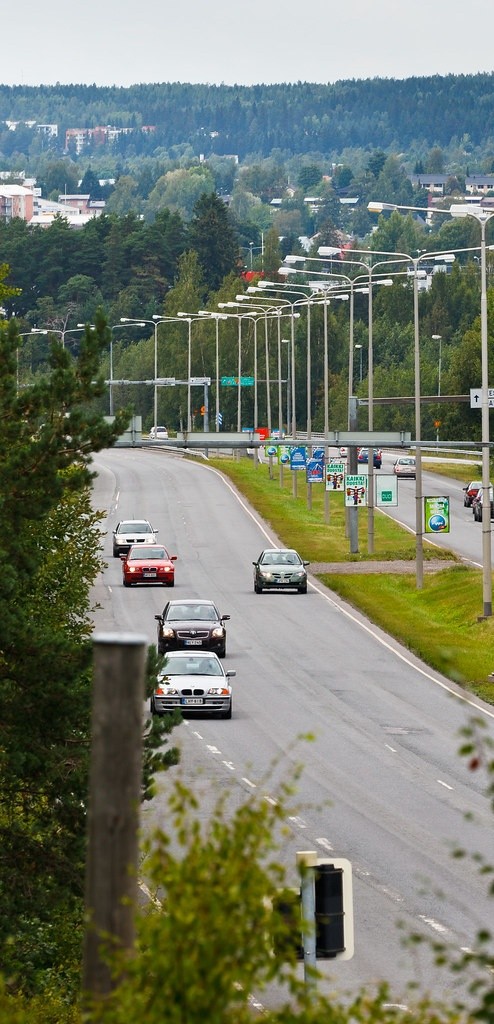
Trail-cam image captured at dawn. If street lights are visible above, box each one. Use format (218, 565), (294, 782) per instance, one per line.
(31, 327), (95, 350)
(119, 317), (192, 439)
(152, 314), (227, 433)
(198, 201), (494, 614)
(3, 330), (48, 398)
(77, 323), (146, 415)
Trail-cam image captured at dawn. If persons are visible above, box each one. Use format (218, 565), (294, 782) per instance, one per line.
(196, 607), (211, 620)
(197, 660), (215, 674)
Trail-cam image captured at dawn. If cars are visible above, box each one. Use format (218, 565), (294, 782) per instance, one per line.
(463, 480), (493, 507)
(471, 487), (494, 522)
(271, 430), (286, 440)
(112, 520), (159, 558)
(393, 457), (416, 480)
(148, 426), (168, 439)
(251, 549), (310, 595)
(339, 447), (361, 458)
(151, 649), (236, 720)
(121, 544), (177, 586)
(358, 448), (382, 469)
(153, 599), (231, 660)
